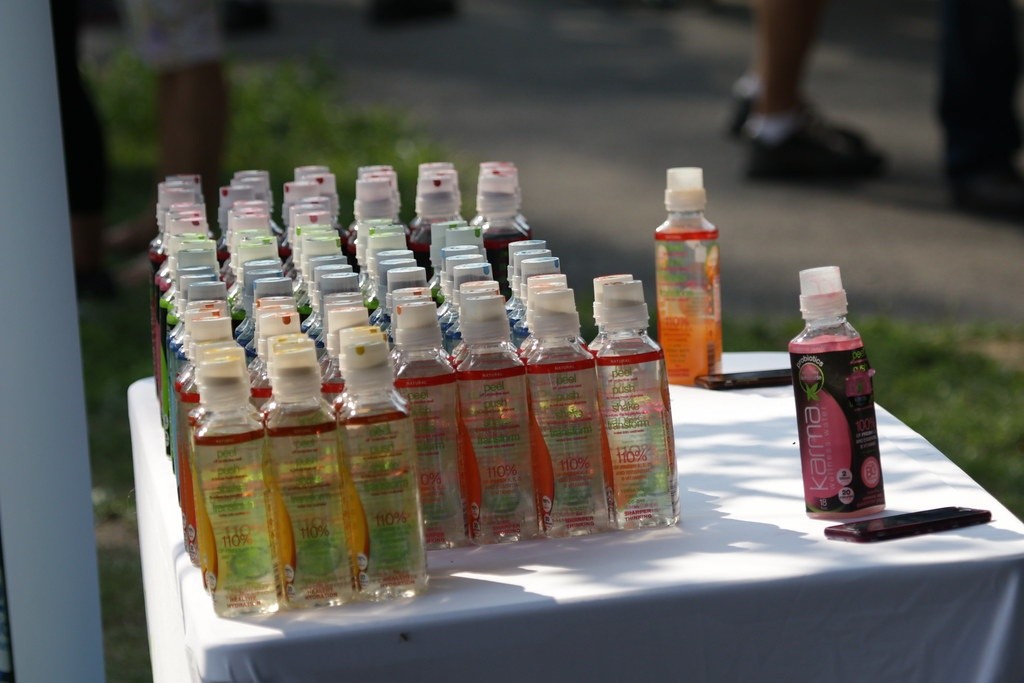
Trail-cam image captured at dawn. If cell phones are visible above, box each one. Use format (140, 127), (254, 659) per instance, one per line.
(825, 507), (991, 543)
(695, 369), (793, 390)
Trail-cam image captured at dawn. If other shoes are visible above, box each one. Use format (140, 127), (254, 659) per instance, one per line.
(728, 100), (885, 186)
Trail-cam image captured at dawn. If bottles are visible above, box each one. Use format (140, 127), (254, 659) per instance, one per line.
(788, 266), (884, 520)
(150, 162), (721, 618)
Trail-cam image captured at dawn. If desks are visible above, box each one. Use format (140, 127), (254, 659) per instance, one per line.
(125, 350), (1024, 683)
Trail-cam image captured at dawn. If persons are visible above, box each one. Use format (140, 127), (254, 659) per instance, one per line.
(102, 0), (229, 288)
(719, 2), (888, 185)
(936, 1), (1024, 221)
